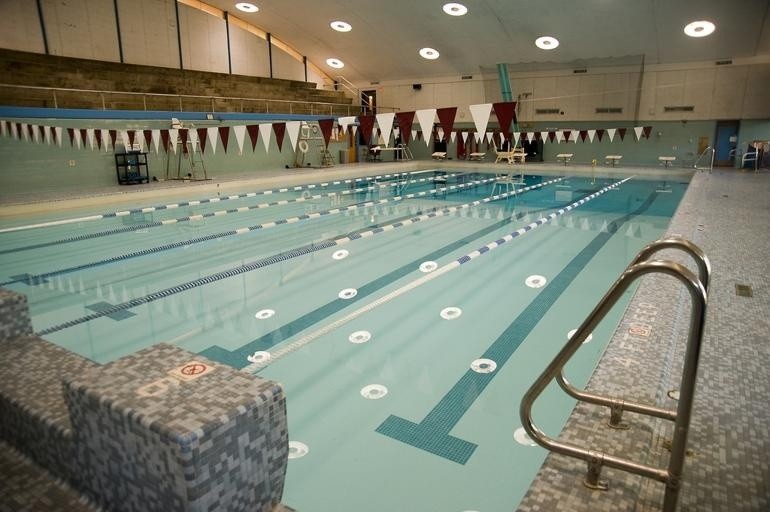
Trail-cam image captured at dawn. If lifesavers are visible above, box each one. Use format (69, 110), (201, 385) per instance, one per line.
(310, 123), (320, 136)
(298, 140), (309, 154)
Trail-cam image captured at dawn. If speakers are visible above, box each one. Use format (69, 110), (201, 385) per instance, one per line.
(413, 84), (421, 89)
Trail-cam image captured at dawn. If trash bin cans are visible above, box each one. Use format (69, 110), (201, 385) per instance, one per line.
(340, 150), (349, 164)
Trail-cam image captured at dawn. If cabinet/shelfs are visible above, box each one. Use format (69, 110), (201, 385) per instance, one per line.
(115, 151), (149, 184)
